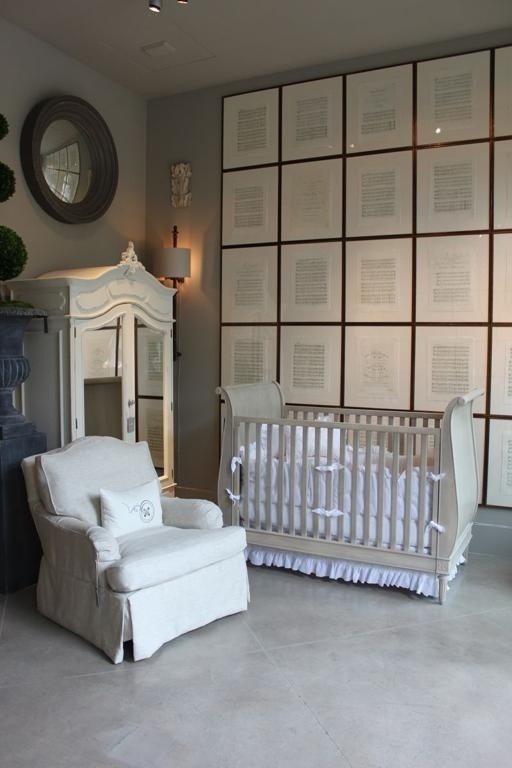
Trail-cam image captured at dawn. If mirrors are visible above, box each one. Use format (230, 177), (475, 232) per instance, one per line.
(19, 93), (120, 226)
(78, 311), (166, 486)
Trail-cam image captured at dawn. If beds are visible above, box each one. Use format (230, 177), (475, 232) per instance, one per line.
(214, 379), (484, 605)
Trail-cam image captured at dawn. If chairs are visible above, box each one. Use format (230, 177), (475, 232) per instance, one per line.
(20, 436), (251, 665)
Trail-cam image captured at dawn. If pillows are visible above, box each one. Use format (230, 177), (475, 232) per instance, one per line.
(99, 478), (163, 538)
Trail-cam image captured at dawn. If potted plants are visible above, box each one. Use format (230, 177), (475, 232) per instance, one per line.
(0, 127), (47, 594)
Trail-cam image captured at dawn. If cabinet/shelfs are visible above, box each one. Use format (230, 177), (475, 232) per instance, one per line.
(0, 240), (177, 498)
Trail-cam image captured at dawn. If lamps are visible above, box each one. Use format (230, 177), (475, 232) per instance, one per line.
(149, 0), (188, 12)
(148, 226), (193, 359)
